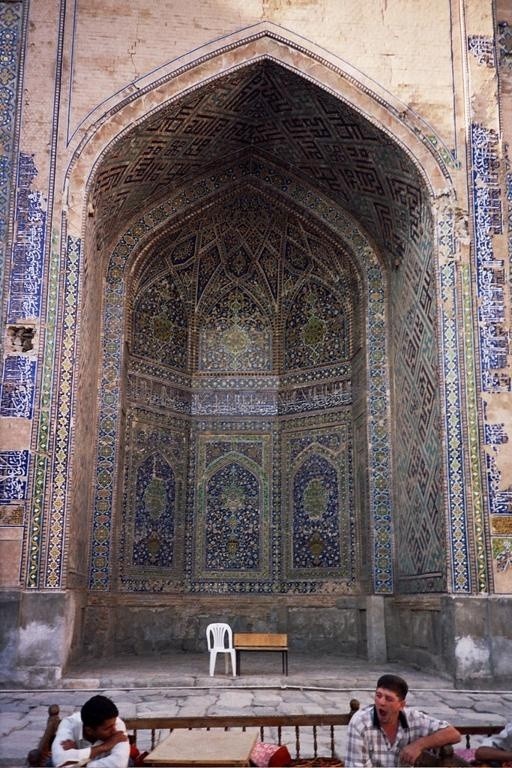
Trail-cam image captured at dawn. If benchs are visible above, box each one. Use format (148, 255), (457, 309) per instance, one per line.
(231, 630), (291, 677)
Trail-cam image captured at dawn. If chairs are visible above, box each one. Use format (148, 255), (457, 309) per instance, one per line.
(21, 722), (512, 765)
(205, 621), (236, 677)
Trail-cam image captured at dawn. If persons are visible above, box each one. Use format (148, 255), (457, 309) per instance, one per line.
(342, 674), (462, 768)
(51, 694), (131, 768)
(473, 718), (512, 768)
